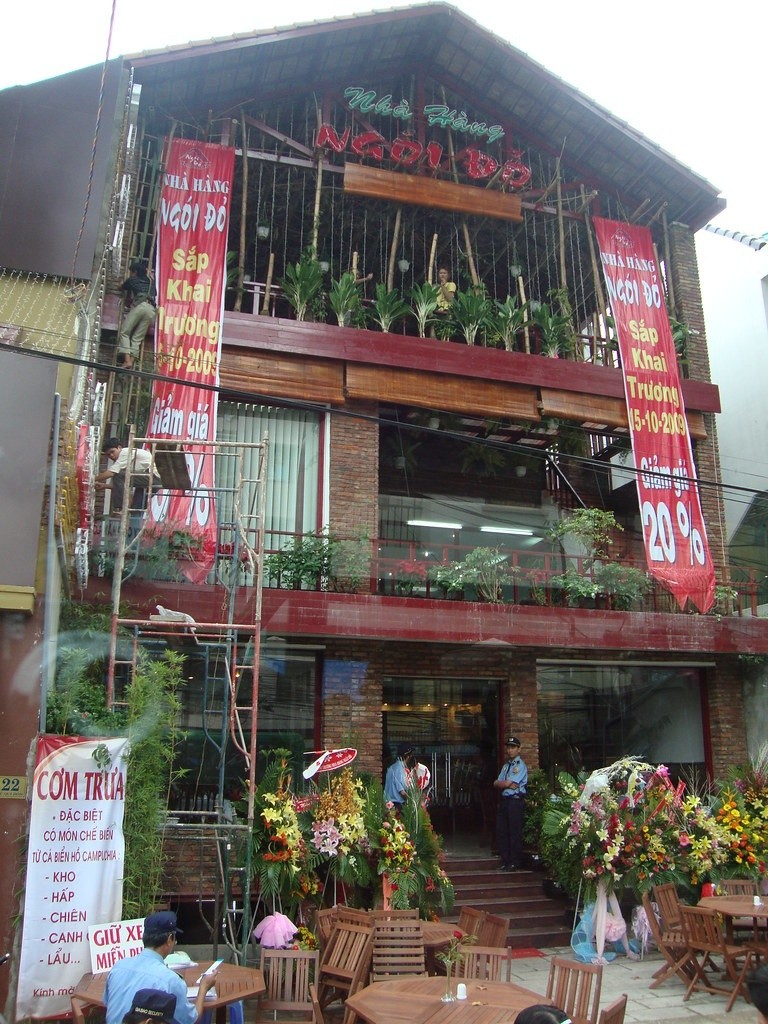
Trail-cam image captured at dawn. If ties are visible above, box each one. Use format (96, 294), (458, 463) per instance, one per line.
(505, 764), (510, 780)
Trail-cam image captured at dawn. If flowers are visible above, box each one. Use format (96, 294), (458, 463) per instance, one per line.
(543, 743), (768, 906)
(230, 730), (478, 984)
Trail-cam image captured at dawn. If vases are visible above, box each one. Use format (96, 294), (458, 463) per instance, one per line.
(440, 962), (456, 1002)
(509, 265), (521, 277)
(398, 259), (409, 273)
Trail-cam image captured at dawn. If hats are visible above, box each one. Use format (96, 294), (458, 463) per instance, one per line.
(505, 737), (520, 748)
(144, 911), (183, 933)
(130, 988), (181, 1024)
(398, 742), (415, 756)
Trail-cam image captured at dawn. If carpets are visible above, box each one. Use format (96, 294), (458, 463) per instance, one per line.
(478, 947), (548, 963)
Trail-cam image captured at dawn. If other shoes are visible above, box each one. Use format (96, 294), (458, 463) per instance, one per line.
(496, 864), (518, 872)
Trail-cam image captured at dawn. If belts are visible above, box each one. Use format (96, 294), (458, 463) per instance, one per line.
(507, 795), (520, 799)
(145, 299), (154, 306)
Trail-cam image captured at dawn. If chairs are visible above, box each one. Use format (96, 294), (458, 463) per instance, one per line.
(71, 997), (85, 1024)
(255, 903), (628, 1024)
(642, 879), (768, 1012)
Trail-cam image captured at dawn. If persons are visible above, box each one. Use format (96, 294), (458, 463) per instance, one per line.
(121, 989), (182, 1024)
(102, 912), (219, 1024)
(94, 437), (162, 517)
(117, 262), (158, 368)
(745, 965), (768, 1024)
(430, 266), (457, 342)
(493, 736), (528, 872)
(383, 744), (415, 818)
(347, 255), (374, 328)
(515, 1006), (570, 1024)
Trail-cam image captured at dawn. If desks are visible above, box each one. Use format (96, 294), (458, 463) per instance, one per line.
(73, 960), (266, 1024)
(697, 894), (768, 981)
(421, 920), (468, 974)
(344, 975), (553, 1024)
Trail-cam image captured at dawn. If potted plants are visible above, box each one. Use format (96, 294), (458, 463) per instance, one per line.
(521, 767), (587, 929)
(318, 254), (337, 273)
(257, 220), (270, 240)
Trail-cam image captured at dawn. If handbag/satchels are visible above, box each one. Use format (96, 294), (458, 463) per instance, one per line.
(132, 292), (147, 307)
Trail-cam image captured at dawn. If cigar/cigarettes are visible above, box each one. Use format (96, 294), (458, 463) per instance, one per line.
(201, 973), (224, 976)
